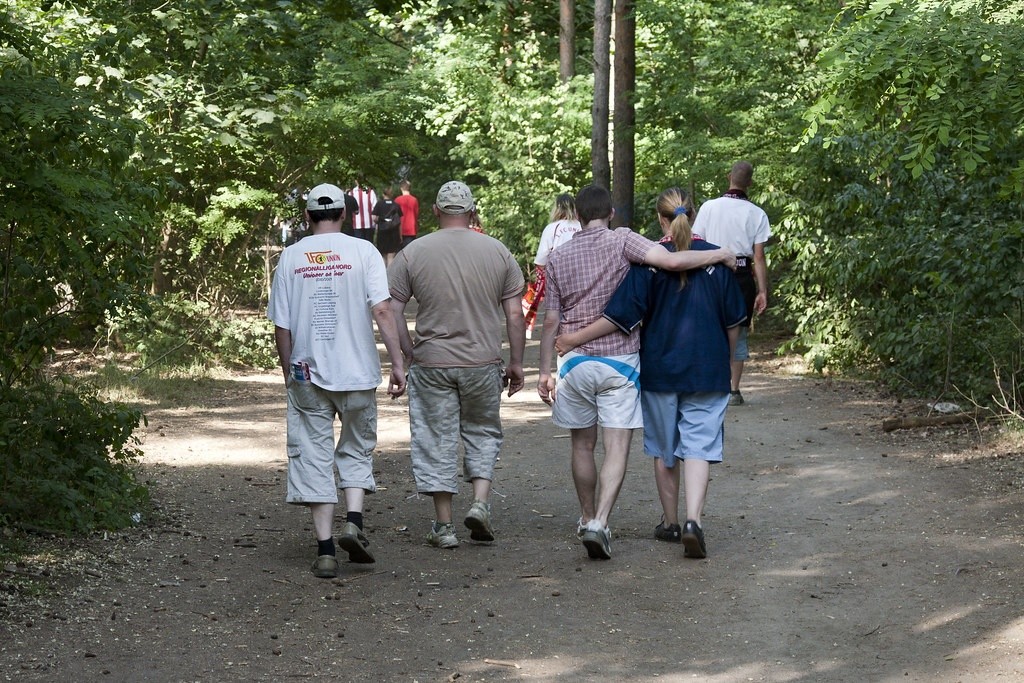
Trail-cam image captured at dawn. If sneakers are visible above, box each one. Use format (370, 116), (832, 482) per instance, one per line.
(427, 520), (459, 548)
(576, 516), (593, 541)
(681, 520), (707, 559)
(465, 501), (495, 540)
(583, 520), (612, 560)
(655, 521), (681, 543)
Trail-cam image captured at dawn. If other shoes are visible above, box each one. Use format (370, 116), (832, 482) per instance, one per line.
(339, 522), (376, 564)
(728, 392), (744, 406)
(312, 555), (338, 577)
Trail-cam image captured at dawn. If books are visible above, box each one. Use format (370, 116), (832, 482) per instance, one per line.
(291, 361), (311, 380)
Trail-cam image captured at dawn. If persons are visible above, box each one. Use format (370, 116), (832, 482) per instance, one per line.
(537, 186), (738, 561)
(370, 186), (404, 270)
(347, 172), (378, 244)
(266, 182), (405, 578)
(554, 187), (748, 558)
(392, 180), (419, 249)
(340, 186), (359, 236)
(534, 194), (583, 271)
(690, 162), (771, 406)
(386, 181), (525, 548)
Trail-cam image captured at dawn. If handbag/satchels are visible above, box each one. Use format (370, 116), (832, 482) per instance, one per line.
(378, 205), (401, 233)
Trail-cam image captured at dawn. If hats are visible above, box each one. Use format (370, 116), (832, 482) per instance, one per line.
(436, 181), (473, 215)
(307, 183), (345, 210)
